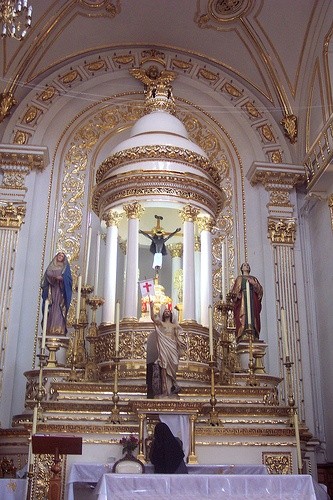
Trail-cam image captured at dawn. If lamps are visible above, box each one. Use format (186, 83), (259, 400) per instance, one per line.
(0, 0), (33, 43)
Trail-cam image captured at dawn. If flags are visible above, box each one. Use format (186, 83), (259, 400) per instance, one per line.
(138, 278), (156, 297)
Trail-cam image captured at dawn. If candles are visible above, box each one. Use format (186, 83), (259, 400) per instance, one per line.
(279, 305), (295, 365)
(26, 405), (39, 478)
(34, 297), (51, 355)
(243, 279), (255, 332)
(292, 410), (305, 475)
(73, 274), (83, 326)
(112, 298), (124, 359)
(206, 305), (216, 361)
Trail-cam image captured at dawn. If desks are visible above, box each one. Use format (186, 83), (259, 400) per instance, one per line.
(65, 463), (269, 500)
(91, 472), (328, 500)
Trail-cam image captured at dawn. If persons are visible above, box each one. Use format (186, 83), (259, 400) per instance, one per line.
(139, 227), (182, 273)
(149, 299), (187, 396)
(40, 252), (73, 335)
(145, 64), (159, 79)
(226, 263), (264, 342)
(149, 423), (189, 474)
